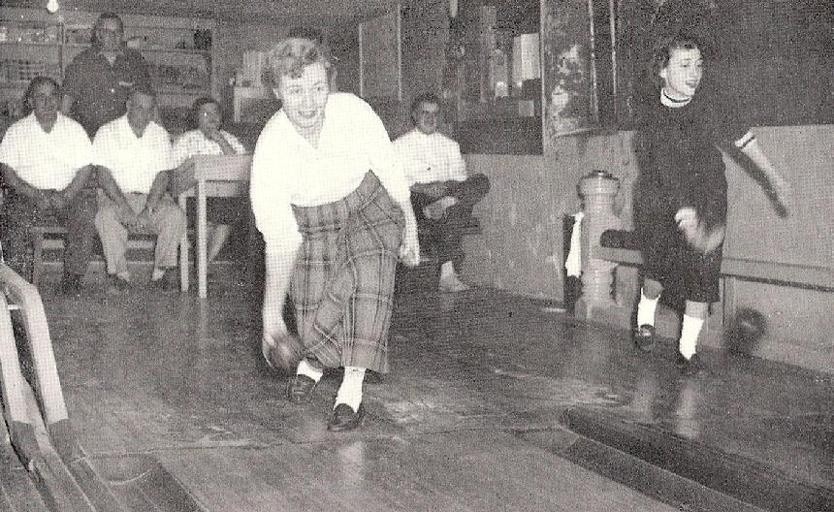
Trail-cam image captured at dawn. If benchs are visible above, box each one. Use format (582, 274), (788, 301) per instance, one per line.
(30, 225), (236, 289)
(578, 171), (834, 376)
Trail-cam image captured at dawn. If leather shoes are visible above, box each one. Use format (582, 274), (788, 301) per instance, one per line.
(287, 374), (317, 403)
(57, 279), (84, 299)
(104, 278), (130, 296)
(148, 274), (174, 292)
(327, 402), (365, 433)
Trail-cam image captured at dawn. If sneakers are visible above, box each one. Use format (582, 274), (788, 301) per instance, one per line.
(635, 323), (658, 351)
(422, 201), (445, 220)
(439, 273), (470, 292)
(676, 350), (713, 378)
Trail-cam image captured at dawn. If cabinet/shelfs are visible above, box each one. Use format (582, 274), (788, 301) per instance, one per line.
(0, 7), (222, 120)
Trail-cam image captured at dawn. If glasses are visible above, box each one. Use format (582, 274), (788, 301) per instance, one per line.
(200, 111), (219, 117)
(98, 28), (125, 38)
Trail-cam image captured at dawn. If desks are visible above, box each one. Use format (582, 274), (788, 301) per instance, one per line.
(173, 153), (252, 299)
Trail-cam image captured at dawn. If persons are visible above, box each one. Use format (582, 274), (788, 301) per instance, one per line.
(90, 83), (188, 294)
(56, 10), (166, 136)
(1, 75), (96, 297)
(249, 36), (422, 436)
(391, 90), (491, 292)
(166, 95), (253, 281)
(627, 35), (793, 378)
